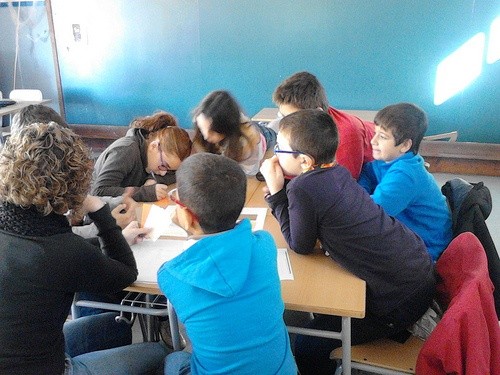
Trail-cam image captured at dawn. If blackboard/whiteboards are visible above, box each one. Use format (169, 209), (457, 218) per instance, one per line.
(46, 0), (500, 159)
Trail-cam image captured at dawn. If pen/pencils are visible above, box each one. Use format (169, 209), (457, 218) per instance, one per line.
(120, 209), (127, 214)
(151, 170), (162, 184)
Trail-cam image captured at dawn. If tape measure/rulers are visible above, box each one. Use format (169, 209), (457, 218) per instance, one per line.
(423, 131), (459, 142)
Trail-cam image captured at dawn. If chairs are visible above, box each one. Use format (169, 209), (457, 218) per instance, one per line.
(2, 89), (43, 135)
(328, 177), (500, 375)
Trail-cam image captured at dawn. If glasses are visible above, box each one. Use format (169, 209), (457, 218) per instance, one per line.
(273, 144), (305, 155)
(277, 111), (284, 119)
(157, 150), (171, 174)
(167, 188), (199, 222)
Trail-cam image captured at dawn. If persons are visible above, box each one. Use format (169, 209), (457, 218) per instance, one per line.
(0, 121), (170, 375)
(7, 72), (378, 319)
(356, 104), (454, 262)
(157, 152), (300, 375)
(259, 111), (435, 375)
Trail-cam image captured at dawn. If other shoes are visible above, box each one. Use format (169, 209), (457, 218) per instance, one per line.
(159, 319), (187, 351)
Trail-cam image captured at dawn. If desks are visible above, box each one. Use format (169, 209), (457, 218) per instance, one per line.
(70, 107), (381, 375)
(0, 99), (53, 143)
